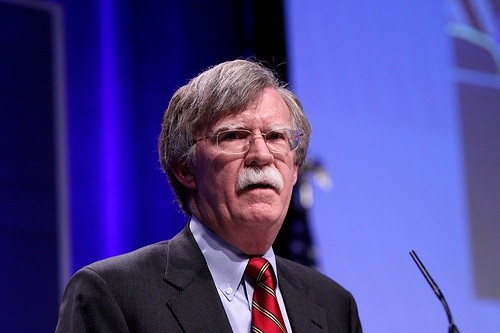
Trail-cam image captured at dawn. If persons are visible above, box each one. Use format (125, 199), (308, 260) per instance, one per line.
(53, 59), (364, 333)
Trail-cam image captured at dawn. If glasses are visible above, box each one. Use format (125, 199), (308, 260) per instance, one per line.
(192, 125), (304, 155)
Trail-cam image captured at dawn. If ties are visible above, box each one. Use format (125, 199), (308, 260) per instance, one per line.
(247, 257), (289, 333)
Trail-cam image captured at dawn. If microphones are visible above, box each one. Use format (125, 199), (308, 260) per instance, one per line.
(409, 249), (461, 333)
